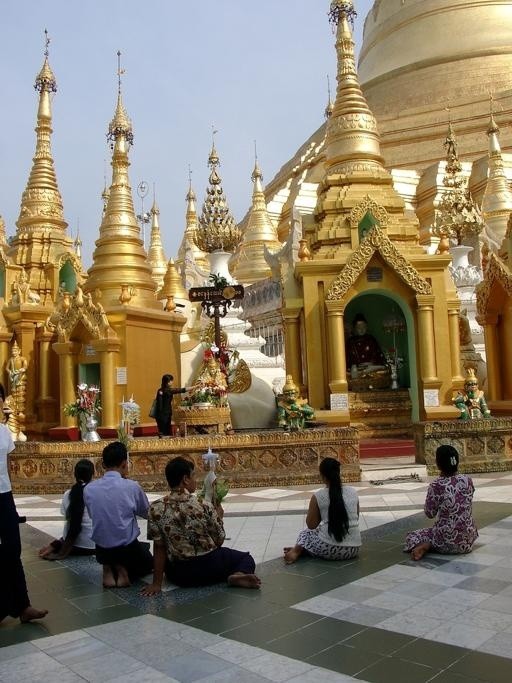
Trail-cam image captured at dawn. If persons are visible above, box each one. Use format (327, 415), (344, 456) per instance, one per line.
(401, 444), (478, 560)
(449, 371), (491, 419)
(139, 455), (262, 597)
(274, 373), (315, 435)
(0, 384), (50, 626)
(5, 339), (29, 416)
(191, 357), (230, 407)
(82, 441), (155, 587)
(154, 373), (199, 436)
(281, 456), (362, 566)
(10, 267), (40, 304)
(40, 458), (96, 561)
(345, 315), (389, 377)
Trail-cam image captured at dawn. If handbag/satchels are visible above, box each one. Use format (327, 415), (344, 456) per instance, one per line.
(149, 399), (157, 419)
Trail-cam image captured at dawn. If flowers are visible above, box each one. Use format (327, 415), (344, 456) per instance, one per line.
(388, 346), (396, 362)
(62, 383), (103, 432)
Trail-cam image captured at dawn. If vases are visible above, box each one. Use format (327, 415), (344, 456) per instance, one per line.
(83, 416), (101, 442)
